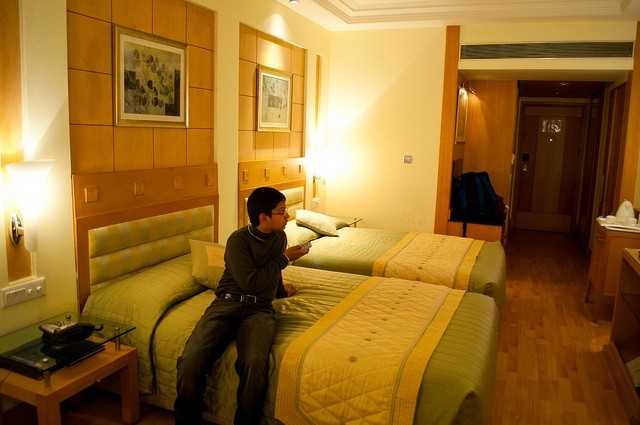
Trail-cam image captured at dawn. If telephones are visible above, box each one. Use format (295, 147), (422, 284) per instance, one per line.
(39, 321), (95, 343)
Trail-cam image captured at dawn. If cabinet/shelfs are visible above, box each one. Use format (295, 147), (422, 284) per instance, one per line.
(602, 248), (639, 425)
(447, 214), (506, 248)
(585, 218), (640, 324)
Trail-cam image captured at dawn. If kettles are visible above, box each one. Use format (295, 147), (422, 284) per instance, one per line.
(615, 199), (635, 224)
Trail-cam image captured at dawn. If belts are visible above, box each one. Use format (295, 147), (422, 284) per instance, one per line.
(216, 293), (266, 304)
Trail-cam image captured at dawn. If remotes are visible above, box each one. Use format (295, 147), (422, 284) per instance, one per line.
(300, 242), (312, 250)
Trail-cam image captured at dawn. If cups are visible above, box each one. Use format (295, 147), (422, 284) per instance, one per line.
(628, 218), (637, 226)
(605, 215), (616, 224)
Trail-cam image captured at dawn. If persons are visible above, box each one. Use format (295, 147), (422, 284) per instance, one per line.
(174, 187), (309, 425)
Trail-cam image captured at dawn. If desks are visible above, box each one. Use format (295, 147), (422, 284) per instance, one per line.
(1, 310), (141, 425)
(320, 211), (364, 228)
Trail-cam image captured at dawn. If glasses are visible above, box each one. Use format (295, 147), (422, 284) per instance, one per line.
(265, 208), (288, 216)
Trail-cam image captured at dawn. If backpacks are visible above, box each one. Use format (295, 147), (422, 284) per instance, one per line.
(452, 172), (506, 237)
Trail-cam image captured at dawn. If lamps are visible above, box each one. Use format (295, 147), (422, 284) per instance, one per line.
(6, 169), (55, 254)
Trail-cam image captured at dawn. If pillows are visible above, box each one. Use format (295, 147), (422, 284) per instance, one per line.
(187, 238), (227, 291)
(295, 208), (339, 237)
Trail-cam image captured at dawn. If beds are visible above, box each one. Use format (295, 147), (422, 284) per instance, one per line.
(240, 176), (508, 312)
(77, 195), (500, 425)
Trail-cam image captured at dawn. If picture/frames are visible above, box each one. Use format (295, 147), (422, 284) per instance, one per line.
(111, 23), (191, 129)
(455, 85), (470, 146)
(256, 62), (293, 132)
